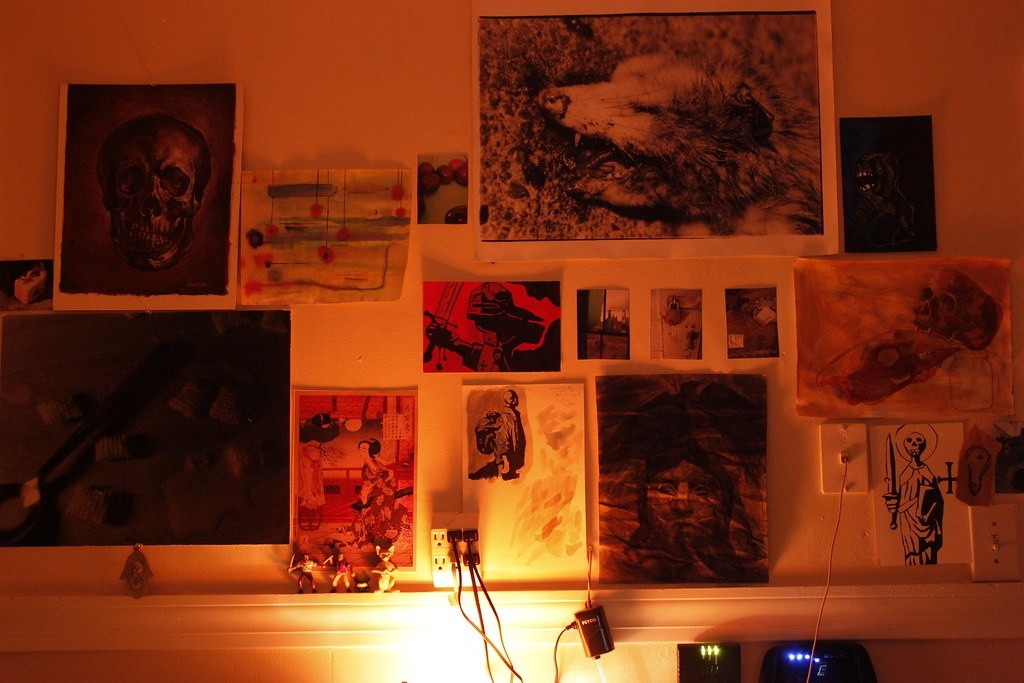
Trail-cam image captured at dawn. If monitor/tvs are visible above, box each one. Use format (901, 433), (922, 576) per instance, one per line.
(676, 642), (742, 683)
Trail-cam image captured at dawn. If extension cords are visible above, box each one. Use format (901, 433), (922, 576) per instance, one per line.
(431, 528), (482, 589)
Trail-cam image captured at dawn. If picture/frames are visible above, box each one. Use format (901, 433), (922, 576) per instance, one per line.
(576, 287), (632, 362)
(724, 285), (781, 360)
(417, 152), (469, 225)
(236, 169), (415, 307)
(961, 423), (1024, 495)
(789, 256), (1017, 425)
(1, 309), (291, 547)
(1, 258), (54, 310)
(649, 288), (704, 360)
(594, 372), (772, 587)
(421, 279), (563, 374)
(293, 387), (419, 569)
(51, 79), (247, 311)
(468, 0), (840, 267)
(458, 381), (590, 582)
(837, 114), (938, 254)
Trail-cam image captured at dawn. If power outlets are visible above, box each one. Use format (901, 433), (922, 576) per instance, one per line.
(430, 528), (483, 589)
(967, 501), (1022, 582)
(818, 421), (873, 495)
